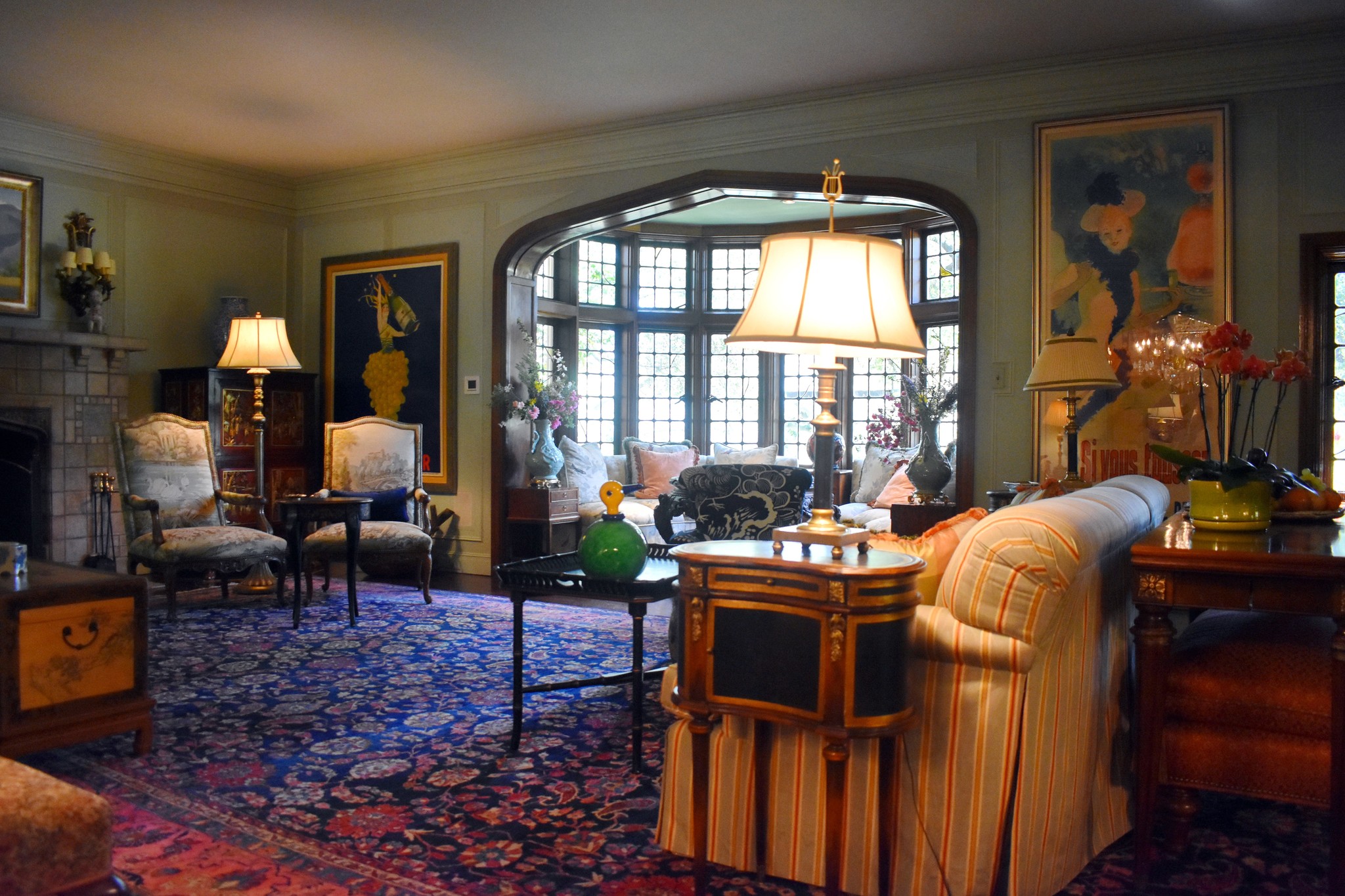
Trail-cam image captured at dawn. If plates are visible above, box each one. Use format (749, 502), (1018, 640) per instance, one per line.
(1272, 508), (1345, 519)
(1003, 481), (1039, 492)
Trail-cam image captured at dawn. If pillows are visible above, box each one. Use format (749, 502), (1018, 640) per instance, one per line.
(850, 442), (923, 509)
(558, 435), (701, 505)
(849, 506), (988, 608)
(712, 442), (779, 465)
(328, 487), (410, 524)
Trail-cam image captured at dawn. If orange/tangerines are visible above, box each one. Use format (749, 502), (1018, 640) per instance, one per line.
(1281, 487), (1342, 512)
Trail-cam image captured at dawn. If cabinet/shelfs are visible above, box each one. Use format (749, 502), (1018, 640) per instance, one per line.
(158, 366), (319, 579)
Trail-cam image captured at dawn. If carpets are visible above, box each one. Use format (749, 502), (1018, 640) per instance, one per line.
(17, 577), (1331, 896)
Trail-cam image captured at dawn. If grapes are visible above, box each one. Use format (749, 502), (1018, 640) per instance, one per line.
(1301, 468), (1327, 490)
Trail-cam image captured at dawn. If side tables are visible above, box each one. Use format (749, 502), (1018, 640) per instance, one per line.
(666, 540), (927, 896)
(508, 487), (580, 556)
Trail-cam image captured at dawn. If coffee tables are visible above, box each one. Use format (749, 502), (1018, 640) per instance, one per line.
(493, 544), (684, 768)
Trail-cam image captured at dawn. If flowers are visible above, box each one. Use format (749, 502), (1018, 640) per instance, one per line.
(1149, 323), (1311, 493)
(486, 318), (582, 430)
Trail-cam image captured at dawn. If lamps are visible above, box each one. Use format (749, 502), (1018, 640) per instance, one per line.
(55, 247), (117, 317)
(1022, 328), (1123, 490)
(722, 159), (927, 563)
(216, 312), (302, 596)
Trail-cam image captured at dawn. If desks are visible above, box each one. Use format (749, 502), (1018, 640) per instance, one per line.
(279, 497), (374, 630)
(1132, 507), (1345, 896)
(0, 559), (156, 760)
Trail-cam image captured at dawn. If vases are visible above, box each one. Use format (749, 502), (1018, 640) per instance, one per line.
(525, 419), (565, 488)
(1186, 478), (1273, 530)
(211, 296), (252, 362)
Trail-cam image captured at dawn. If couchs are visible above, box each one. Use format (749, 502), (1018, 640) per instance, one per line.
(576, 455), (799, 550)
(661, 474), (1170, 896)
(835, 458), (891, 534)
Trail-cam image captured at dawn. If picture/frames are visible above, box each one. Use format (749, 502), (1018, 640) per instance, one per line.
(319, 242), (461, 496)
(1032, 100), (1237, 487)
(0, 168), (44, 319)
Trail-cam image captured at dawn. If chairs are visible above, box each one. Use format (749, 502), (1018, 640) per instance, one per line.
(302, 416), (433, 605)
(654, 463), (813, 545)
(109, 412), (287, 621)
